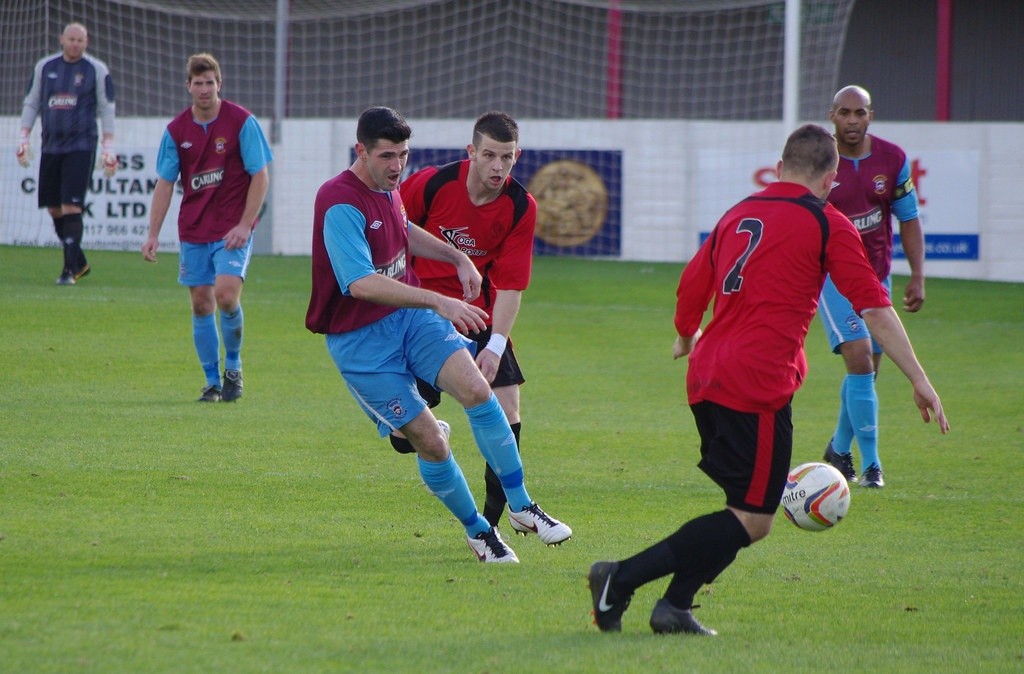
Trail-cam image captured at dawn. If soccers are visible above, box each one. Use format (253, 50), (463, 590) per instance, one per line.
(779, 462), (849, 531)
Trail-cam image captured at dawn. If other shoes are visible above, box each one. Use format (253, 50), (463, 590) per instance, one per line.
(423, 420), (450, 495)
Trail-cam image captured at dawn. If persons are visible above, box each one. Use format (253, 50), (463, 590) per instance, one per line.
(303, 105), (572, 565)
(16, 25), (119, 285)
(819, 86), (927, 488)
(143, 54), (271, 402)
(398, 110), (536, 528)
(584, 124), (950, 637)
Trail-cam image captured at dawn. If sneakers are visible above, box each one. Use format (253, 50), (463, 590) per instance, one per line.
(587, 561), (634, 631)
(221, 368), (243, 401)
(199, 384), (222, 401)
(650, 599), (717, 635)
(860, 463), (885, 488)
(467, 524), (519, 563)
(823, 437), (860, 484)
(56, 261), (90, 284)
(507, 500), (573, 548)
